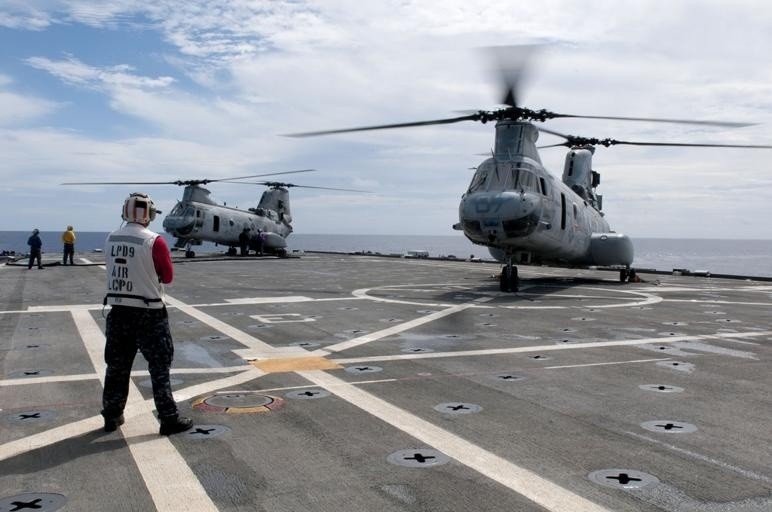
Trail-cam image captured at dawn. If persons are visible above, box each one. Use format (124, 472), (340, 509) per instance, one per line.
(62, 225), (77, 265)
(99, 192), (194, 435)
(27, 228), (45, 269)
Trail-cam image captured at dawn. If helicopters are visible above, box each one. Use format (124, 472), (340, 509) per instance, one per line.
(60, 168), (374, 260)
(277, 41), (772, 294)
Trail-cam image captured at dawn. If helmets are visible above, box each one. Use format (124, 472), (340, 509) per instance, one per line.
(121, 192), (157, 224)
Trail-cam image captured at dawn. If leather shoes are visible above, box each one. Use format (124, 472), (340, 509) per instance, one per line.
(158, 415), (193, 436)
(103, 413), (125, 433)
